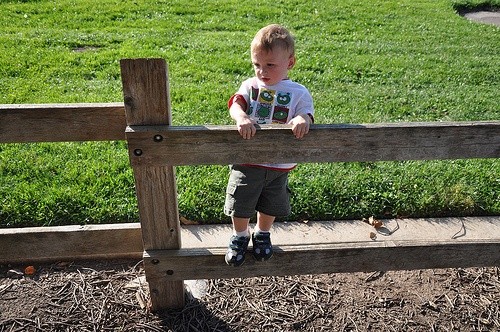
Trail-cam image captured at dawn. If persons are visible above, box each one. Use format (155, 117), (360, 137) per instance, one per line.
(222, 23), (314, 269)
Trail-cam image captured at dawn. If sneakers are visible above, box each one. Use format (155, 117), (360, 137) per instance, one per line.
(252, 231), (273, 263)
(225, 228), (251, 268)
